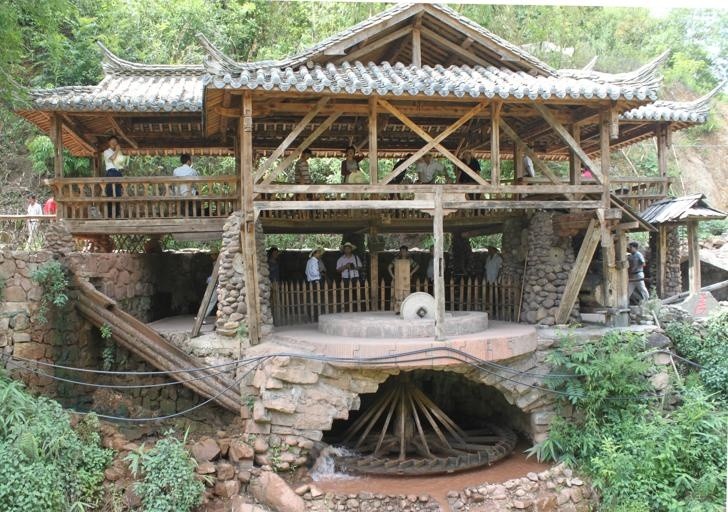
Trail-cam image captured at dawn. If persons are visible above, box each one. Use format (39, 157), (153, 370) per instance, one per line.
(267, 246), (284, 314)
(415, 150), (443, 185)
(303, 245), (326, 320)
(103, 136), (126, 217)
(513, 151), (536, 200)
(453, 149), (486, 216)
(581, 162), (593, 177)
(340, 145), (366, 215)
(334, 242), (363, 313)
(626, 240), (650, 307)
(25, 193), (43, 252)
(287, 146), (314, 217)
(172, 154), (200, 215)
(387, 152), (414, 219)
(483, 244), (503, 315)
(425, 244), (446, 298)
(41, 194), (56, 225)
(387, 245), (421, 280)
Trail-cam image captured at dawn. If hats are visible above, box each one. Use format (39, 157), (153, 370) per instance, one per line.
(308, 246), (325, 259)
(206, 246), (219, 255)
(348, 170), (365, 184)
(338, 241), (357, 252)
(113, 150), (126, 169)
(484, 242), (499, 252)
